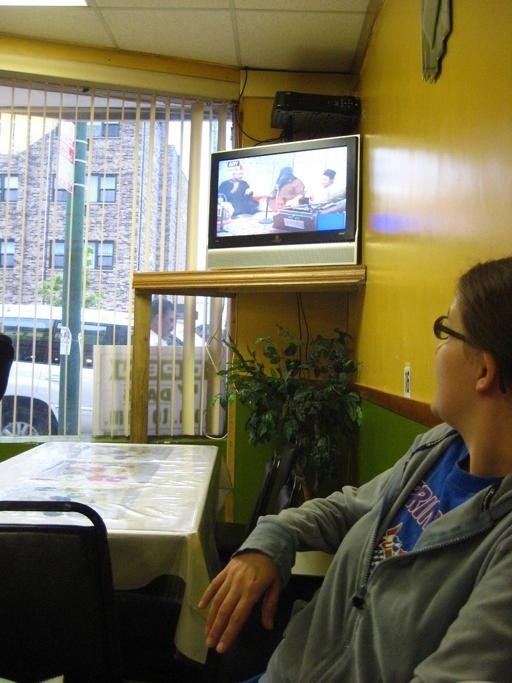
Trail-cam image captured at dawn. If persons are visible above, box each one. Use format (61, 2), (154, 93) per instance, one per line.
(195, 252), (512, 683)
(218, 164), (257, 218)
(149, 297), (184, 345)
(266, 165), (306, 214)
(300, 168), (346, 214)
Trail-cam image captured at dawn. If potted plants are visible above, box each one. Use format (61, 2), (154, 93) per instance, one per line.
(206, 323), (366, 540)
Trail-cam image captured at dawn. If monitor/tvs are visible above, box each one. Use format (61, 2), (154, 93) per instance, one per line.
(205, 132), (363, 270)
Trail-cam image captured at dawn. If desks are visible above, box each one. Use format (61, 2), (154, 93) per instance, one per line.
(0, 440), (234, 683)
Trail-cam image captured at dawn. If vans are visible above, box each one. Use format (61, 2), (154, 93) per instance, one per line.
(0, 301), (207, 441)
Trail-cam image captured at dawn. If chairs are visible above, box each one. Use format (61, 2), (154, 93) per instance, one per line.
(213, 574), (327, 683)
(0, 500), (121, 683)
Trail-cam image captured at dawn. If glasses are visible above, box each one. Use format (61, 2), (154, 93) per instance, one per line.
(434, 316), (505, 395)
